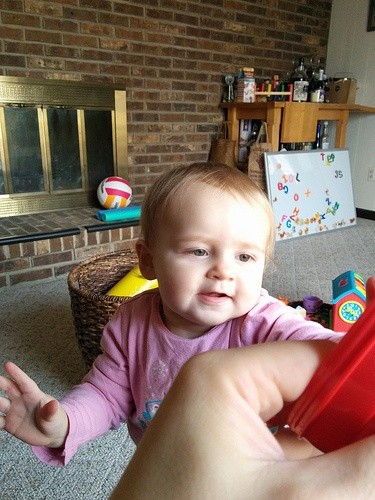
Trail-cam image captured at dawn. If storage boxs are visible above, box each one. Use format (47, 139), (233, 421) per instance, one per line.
(322, 78), (357, 103)
(67, 248), (158, 374)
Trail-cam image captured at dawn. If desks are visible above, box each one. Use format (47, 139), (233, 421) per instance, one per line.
(219, 100), (374, 174)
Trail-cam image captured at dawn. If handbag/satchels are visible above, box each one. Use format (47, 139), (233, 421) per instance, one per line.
(248, 123), (273, 191)
(209, 121), (235, 168)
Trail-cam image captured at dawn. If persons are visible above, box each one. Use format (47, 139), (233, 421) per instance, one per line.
(1, 161), (348, 470)
(109, 339), (375, 500)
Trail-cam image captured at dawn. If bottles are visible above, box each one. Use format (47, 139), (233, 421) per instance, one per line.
(248, 59), (329, 152)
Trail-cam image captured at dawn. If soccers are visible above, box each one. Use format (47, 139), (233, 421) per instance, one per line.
(97, 176), (133, 210)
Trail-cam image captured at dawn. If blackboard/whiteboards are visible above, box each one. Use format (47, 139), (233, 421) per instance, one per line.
(263, 147), (357, 243)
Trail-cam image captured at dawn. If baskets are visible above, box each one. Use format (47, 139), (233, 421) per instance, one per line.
(68, 248), (140, 372)
